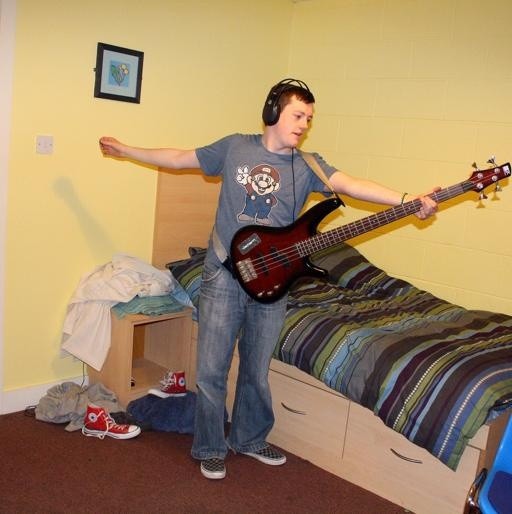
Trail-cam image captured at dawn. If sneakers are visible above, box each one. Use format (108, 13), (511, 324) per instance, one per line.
(200, 458), (226, 480)
(240, 445), (287, 466)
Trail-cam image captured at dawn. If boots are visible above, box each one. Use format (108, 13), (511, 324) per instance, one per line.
(149, 371), (188, 399)
(84, 404), (141, 439)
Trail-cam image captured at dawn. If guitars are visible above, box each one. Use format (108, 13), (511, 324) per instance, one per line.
(230, 157), (509, 302)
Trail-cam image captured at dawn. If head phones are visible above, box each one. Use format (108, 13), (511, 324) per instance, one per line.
(262, 78), (312, 126)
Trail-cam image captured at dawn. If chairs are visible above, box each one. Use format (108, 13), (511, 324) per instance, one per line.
(462, 417), (512, 514)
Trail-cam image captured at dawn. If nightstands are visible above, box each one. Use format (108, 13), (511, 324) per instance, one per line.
(85, 305), (197, 412)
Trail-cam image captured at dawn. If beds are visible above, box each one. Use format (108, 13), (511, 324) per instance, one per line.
(150, 157), (510, 514)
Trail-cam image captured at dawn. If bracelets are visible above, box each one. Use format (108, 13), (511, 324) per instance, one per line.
(400, 190), (408, 206)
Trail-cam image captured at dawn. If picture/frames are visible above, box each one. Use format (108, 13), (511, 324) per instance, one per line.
(93, 40), (145, 104)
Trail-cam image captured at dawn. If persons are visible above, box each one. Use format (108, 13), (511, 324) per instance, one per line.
(98, 76), (442, 479)
